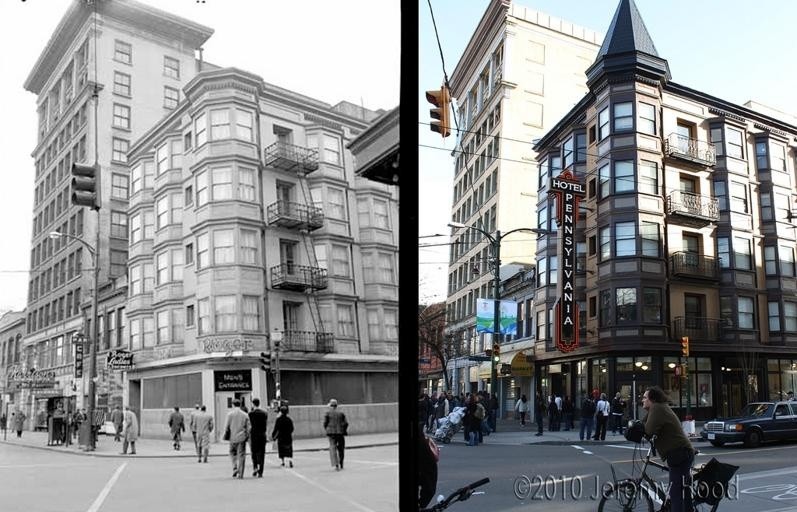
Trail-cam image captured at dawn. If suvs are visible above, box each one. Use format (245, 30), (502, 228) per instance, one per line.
(699, 400), (796, 448)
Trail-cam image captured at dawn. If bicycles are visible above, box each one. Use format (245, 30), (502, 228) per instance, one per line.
(595, 437), (733, 511)
(415, 475), (494, 510)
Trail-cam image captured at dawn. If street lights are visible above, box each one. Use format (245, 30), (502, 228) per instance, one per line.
(51, 231), (98, 451)
(450, 220), (548, 436)
(270, 326), (283, 405)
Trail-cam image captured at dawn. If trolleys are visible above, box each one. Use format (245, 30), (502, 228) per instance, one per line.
(432, 403), (466, 445)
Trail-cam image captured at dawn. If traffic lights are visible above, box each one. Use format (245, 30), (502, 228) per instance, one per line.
(427, 86), (457, 142)
(680, 335), (688, 358)
(491, 342), (501, 366)
(71, 160), (102, 213)
(674, 364), (685, 377)
(260, 350), (275, 375)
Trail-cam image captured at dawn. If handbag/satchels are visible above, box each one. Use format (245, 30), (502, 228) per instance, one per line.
(693, 458), (739, 505)
(623, 420), (644, 444)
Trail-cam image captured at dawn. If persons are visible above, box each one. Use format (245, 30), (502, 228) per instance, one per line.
(167, 406), (185, 450)
(0, 404), (138, 454)
(417, 389), (627, 512)
(190, 398), (294, 480)
(322, 398), (348, 470)
(786, 391), (797, 402)
(699, 392), (709, 406)
(640, 386), (694, 512)
